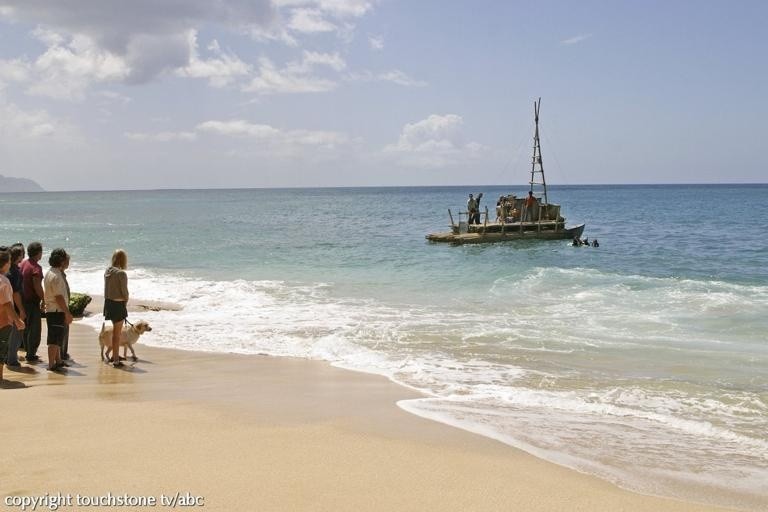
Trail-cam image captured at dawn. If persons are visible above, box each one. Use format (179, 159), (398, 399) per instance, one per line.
(582, 237), (591, 247)
(0, 240), (72, 388)
(591, 238), (599, 247)
(571, 235), (582, 247)
(101, 249), (129, 368)
(466, 193), (477, 224)
(474, 192), (483, 225)
(523, 190), (536, 225)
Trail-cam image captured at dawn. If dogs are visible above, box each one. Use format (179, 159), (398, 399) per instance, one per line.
(99, 320), (152, 362)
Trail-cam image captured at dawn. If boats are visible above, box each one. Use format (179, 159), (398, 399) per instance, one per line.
(422, 94), (589, 248)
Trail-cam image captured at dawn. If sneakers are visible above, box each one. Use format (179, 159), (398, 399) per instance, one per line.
(110, 355), (126, 361)
(113, 362), (123, 368)
(1, 350), (74, 387)
